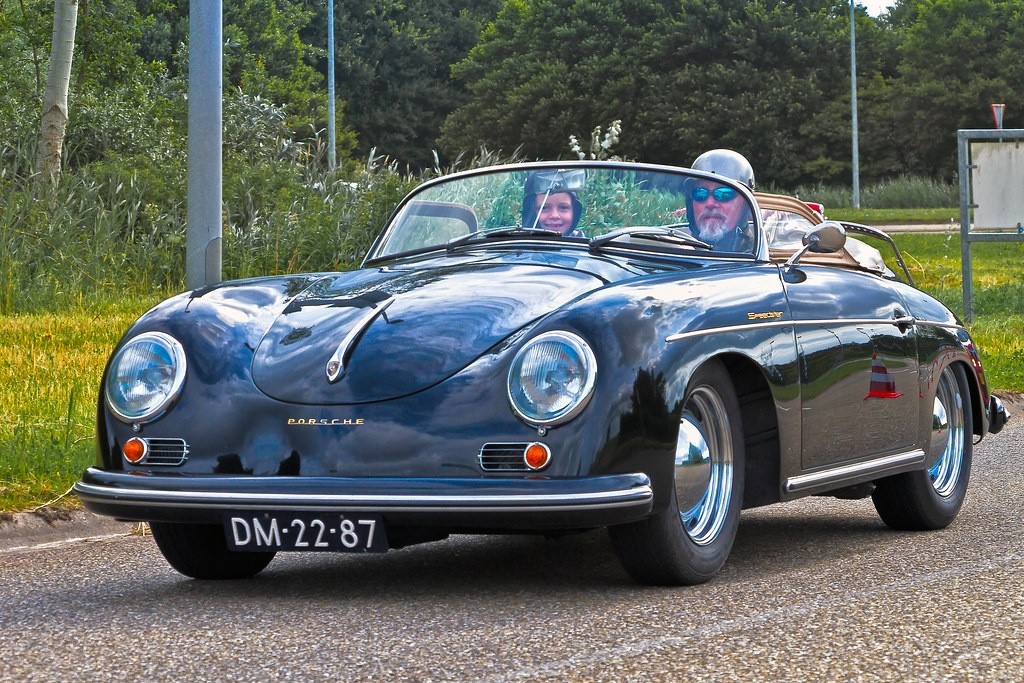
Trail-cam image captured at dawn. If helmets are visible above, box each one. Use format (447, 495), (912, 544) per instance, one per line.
(684, 149), (755, 196)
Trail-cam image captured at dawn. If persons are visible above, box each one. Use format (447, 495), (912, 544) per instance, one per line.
(522, 167), (584, 237)
(683, 149), (755, 253)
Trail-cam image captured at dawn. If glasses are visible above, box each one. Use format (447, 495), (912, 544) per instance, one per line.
(691, 187), (737, 202)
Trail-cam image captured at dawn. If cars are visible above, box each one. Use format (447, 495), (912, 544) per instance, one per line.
(73, 159), (1012, 589)
